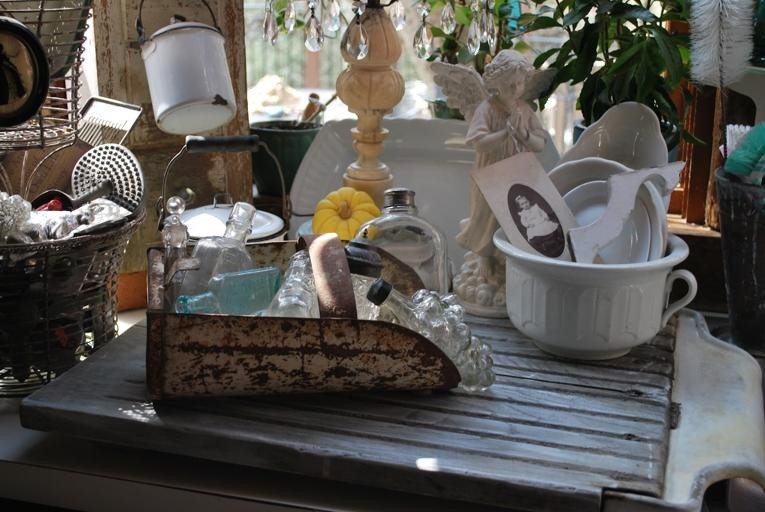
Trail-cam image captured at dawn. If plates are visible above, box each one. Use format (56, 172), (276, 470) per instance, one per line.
(542, 100), (672, 262)
(286, 115), (471, 273)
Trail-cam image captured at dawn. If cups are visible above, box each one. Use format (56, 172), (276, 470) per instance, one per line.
(493, 228), (698, 361)
(249, 119), (324, 198)
(138, 15), (237, 134)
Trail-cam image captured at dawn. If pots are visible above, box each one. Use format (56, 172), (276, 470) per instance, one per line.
(151, 134), (290, 243)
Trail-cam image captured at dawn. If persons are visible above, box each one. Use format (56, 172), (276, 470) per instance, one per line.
(512, 192), (562, 255)
(428, 48), (560, 262)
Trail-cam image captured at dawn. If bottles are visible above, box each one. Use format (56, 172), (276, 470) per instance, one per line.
(366, 278), (496, 394)
(175, 201), (256, 296)
(174, 266), (282, 313)
(352, 188), (448, 295)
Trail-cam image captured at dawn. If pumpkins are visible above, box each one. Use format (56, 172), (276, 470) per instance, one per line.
(312, 187), (381, 241)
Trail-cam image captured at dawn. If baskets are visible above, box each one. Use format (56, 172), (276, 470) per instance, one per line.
(0, 206), (145, 397)
(0, 1), (92, 150)
